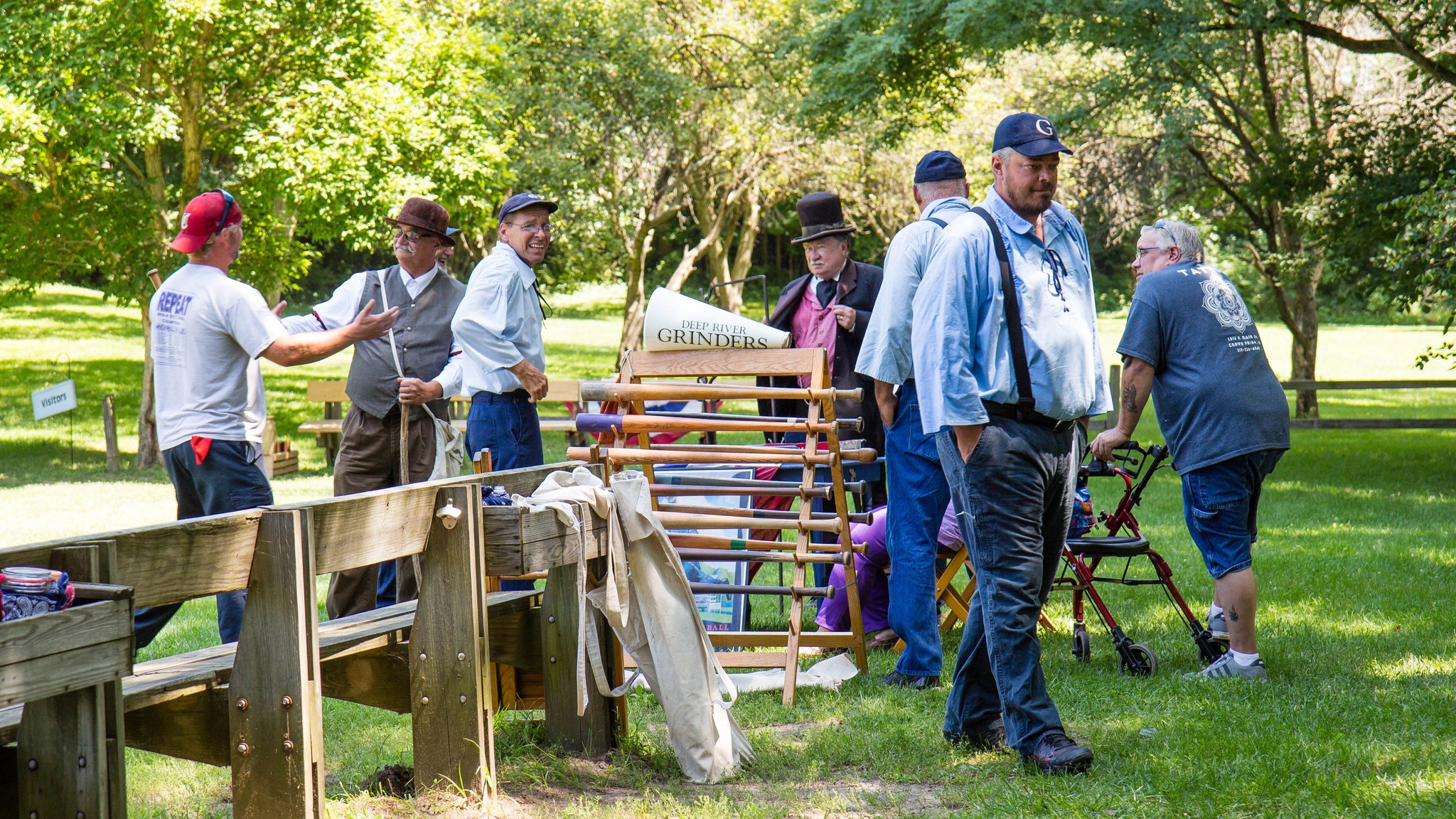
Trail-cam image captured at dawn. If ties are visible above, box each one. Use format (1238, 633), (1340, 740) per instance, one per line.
(815, 278), (838, 310)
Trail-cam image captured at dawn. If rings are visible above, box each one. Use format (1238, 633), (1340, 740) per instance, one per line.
(840, 316), (842, 319)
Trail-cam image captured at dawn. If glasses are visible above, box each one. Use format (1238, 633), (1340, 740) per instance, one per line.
(503, 222), (557, 233)
(1152, 218), (1177, 246)
(1135, 247), (1159, 260)
(391, 226), (439, 243)
(209, 188), (235, 236)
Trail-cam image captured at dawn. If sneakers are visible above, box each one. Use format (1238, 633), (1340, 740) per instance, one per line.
(1183, 649), (1267, 680)
(878, 670), (941, 691)
(943, 726), (1014, 755)
(1205, 608), (1231, 640)
(1022, 734), (1094, 778)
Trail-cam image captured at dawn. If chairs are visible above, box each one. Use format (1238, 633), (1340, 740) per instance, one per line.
(892, 540), (1056, 654)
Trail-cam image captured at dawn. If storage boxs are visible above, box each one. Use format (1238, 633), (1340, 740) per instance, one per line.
(262, 452), (298, 478)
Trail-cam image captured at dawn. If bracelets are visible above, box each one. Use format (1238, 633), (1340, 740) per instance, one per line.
(405, 393), (408, 399)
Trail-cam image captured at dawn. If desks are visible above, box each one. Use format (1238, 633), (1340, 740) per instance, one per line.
(628, 457), (885, 630)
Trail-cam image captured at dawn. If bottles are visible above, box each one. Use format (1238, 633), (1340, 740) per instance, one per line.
(491, 485), (510, 499)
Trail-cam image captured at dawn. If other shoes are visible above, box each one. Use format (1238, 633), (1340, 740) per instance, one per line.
(721, 578), (732, 600)
(865, 628), (900, 649)
(785, 646), (843, 655)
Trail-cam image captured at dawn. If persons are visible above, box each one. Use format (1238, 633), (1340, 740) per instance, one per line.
(1090, 219), (1291, 680)
(913, 112), (1113, 776)
(754, 150), (971, 689)
(451, 193), (558, 591)
(134, 188), (399, 657)
(271, 199), (467, 621)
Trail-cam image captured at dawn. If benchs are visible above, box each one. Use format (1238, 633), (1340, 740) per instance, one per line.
(298, 377), (588, 469)
(0, 444), (618, 818)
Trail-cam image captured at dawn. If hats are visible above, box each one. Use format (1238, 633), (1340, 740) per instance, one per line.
(170, 192), (242, 254)
(384, 197), (457, 247)
(913, 150), (966, 183)
(446, 225), (462, 236)
(991, 112), (1073, 157)
(791, 192), (857, 244)
(498, 193), (558, 225)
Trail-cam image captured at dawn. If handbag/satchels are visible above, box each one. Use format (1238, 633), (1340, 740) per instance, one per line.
(427, 418), (464, 481)
